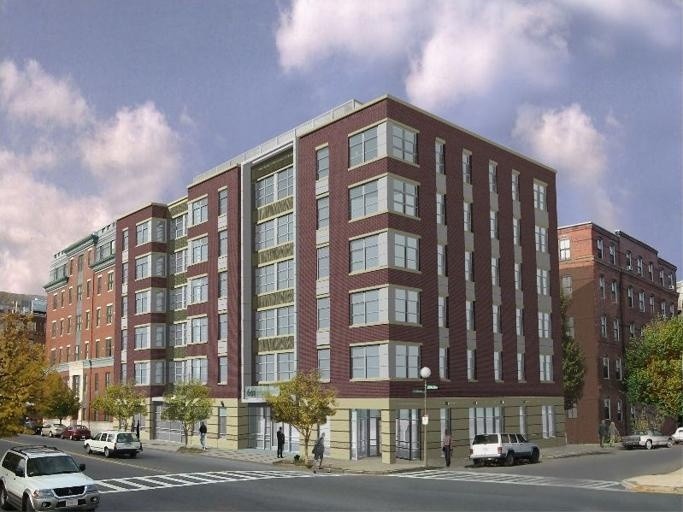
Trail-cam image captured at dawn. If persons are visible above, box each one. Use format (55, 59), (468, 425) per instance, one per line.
(442, 429), (453, 467)
(277, 427), (285, 458)
(199, 422), (207, 450)
(608, 422), (619, 447)
(598, 419), (609, 448)
(312, 432), (325, 469)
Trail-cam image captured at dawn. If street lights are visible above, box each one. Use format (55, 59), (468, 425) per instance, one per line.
(419, 367), (431, 468)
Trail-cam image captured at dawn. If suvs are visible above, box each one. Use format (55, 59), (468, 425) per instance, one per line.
(0, 444), (99, 512)
(83, 430), (142, 457)
(466, 432), (540, 466)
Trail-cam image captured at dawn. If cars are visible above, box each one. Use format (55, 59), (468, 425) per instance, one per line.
(40, 423), (67, 437)
(671, 426), (682, 445)
(22, 419), (44, 435)
(621, 428), (675, 449)
(60, 424), (90, 441)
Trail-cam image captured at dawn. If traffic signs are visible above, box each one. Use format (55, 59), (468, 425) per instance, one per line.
(426, 384), (438, 389)
(412, 389), (424, 393)
